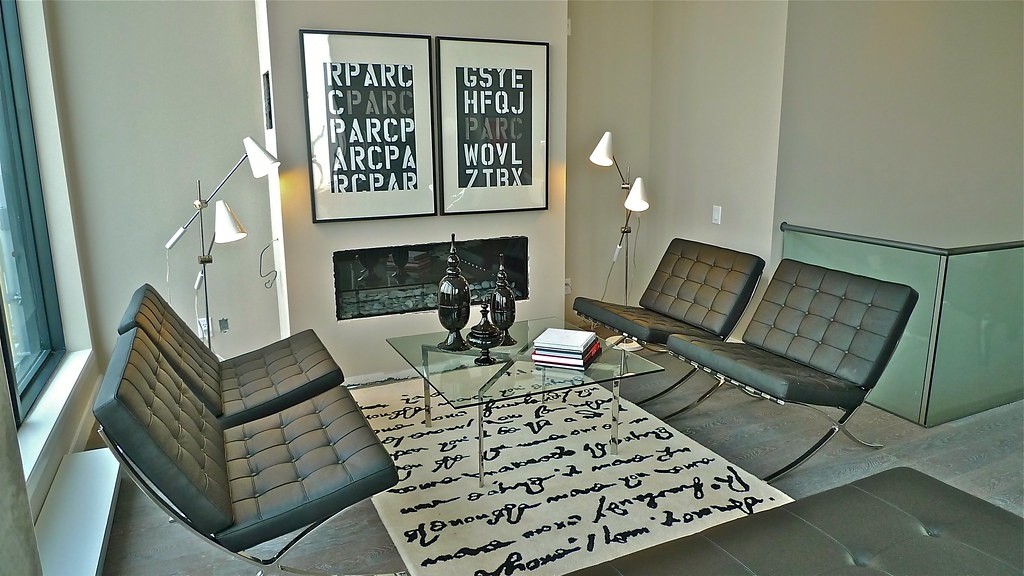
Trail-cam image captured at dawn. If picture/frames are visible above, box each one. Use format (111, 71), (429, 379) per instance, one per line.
(299, 28), (437, 223)
(434, 36), (549, 216)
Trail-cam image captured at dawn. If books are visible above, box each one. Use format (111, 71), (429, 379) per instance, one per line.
(531, 327), (602, 371)
(387, 251), (432, 275)
(532, 353), (603, 382)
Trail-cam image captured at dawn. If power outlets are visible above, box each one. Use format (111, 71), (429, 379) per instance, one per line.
(197, 318), (213, 340)
(565, 278), (572, 295)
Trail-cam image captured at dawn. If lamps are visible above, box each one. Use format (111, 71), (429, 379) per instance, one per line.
(166, 137), (281, 353)
(588, 131), (650, 351)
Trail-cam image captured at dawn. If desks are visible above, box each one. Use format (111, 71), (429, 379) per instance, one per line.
(386, 316), (665, 487)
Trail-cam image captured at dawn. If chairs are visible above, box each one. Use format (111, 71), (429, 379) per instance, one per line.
(93, 285), (406, 576)
(573, 239), (920, 483)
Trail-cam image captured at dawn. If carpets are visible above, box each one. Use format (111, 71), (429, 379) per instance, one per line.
(340, 360), (796, 576)
